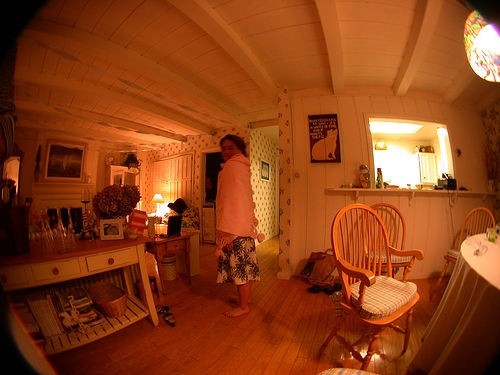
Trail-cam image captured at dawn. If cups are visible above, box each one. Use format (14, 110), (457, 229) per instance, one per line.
(28, 207), (76, 257)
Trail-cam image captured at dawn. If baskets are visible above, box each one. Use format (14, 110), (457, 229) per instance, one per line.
(90, 283), (127, 317)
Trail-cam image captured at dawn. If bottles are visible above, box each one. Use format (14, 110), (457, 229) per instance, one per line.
(359, 164), (370, 188)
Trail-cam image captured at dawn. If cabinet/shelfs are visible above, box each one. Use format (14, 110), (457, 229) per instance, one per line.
(104, 165), (139, 208)
(420, 153), (436, 182)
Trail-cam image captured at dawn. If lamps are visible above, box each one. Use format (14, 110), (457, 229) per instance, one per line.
(150, 194), (164, 214)
(465, 11), (500, 83)
(375, 138), (387, 150)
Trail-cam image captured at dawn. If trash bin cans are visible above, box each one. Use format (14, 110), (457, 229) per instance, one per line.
(159, 253), (177, 280)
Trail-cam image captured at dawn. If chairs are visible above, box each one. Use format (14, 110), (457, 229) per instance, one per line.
(430, 207), (495, 298)
(318, 203), (423, 371)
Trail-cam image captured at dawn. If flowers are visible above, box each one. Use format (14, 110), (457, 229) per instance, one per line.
(92, 183), (140, 218)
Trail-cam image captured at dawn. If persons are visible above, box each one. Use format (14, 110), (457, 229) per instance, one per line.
(216, 135), (265, 316)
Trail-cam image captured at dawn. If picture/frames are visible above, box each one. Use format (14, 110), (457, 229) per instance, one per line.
(261, 159), (271, 181)
(102, 220), (123, 240)
(41, 138), (87, 182)
(308, 112), (343, 166)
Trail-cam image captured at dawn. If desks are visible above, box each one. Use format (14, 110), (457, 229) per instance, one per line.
(148, 232), (201, 287)
(405, 227), (500, 375)
(0, 235), (160, 374)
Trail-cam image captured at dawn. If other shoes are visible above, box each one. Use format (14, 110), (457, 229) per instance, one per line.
(159, 305), (170, 313)
(163, 313), (176, 327)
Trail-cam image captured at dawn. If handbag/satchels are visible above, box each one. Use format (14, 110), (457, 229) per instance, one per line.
(300, 249), (337, 288)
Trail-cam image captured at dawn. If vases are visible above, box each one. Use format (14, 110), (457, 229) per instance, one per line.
(111, 216), (126, 230)
